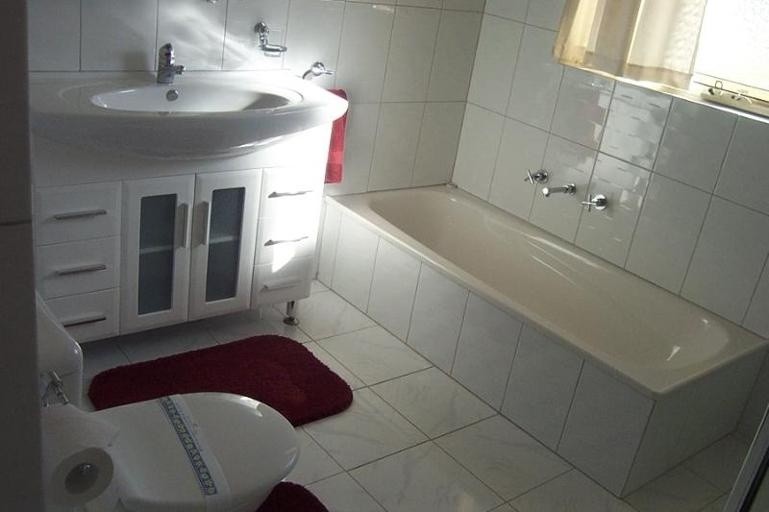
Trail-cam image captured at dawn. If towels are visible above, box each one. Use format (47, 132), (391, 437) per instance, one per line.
(322, 88), (348, 183)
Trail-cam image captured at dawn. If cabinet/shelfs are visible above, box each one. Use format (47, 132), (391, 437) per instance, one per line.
(120, 165), (264, 337)
(250, 161), (327, 310)
(32, 180), (121, 346)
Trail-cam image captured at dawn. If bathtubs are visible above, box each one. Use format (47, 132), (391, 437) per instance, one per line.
(325, 182), (769, 401)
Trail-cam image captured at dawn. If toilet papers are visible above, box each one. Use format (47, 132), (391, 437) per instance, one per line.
(39, 403), (121, 512)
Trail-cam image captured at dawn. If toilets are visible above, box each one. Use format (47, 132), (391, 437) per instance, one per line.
(34, 292), (301, 512)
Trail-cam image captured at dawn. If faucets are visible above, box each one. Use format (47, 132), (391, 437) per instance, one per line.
(542, 183), (576, 196)
(157, 42), (186, 84)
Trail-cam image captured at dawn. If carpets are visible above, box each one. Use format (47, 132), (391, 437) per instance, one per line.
(256, 478), (329, 512)
(89, 329), (355, 425)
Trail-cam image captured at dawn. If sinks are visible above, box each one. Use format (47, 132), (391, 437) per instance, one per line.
(59, 76), (303, 117)
